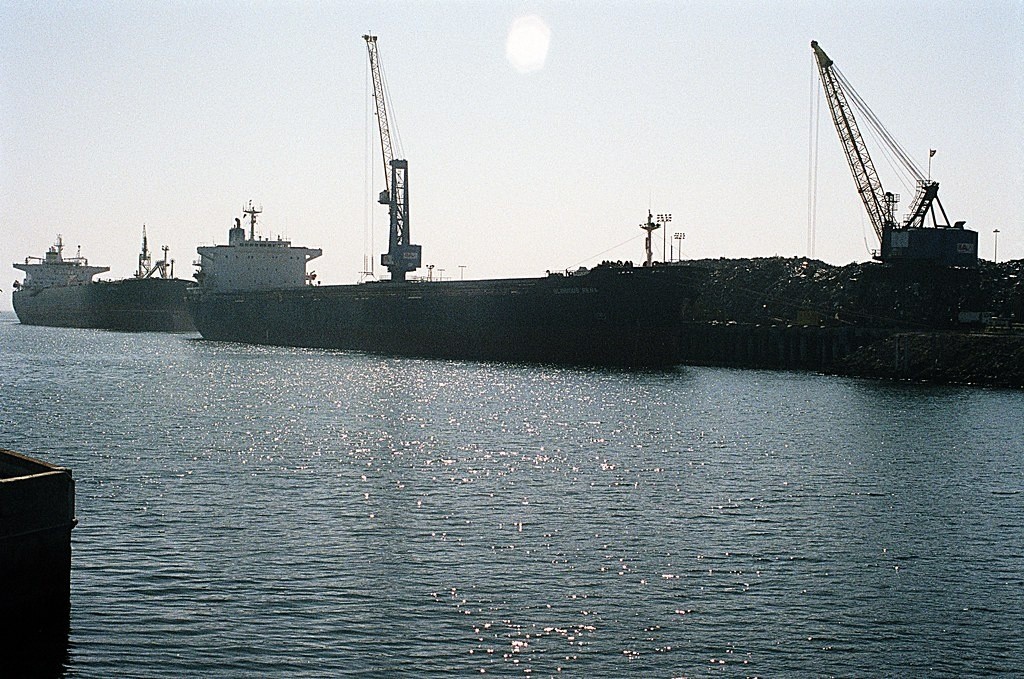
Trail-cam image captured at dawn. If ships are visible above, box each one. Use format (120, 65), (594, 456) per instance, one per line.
(190, 200), (719, 369)
(11, 233), (197, 340)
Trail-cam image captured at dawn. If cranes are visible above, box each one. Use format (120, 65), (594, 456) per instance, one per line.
(812, 41), (979, 267)
(365, 31), (422, 280)
